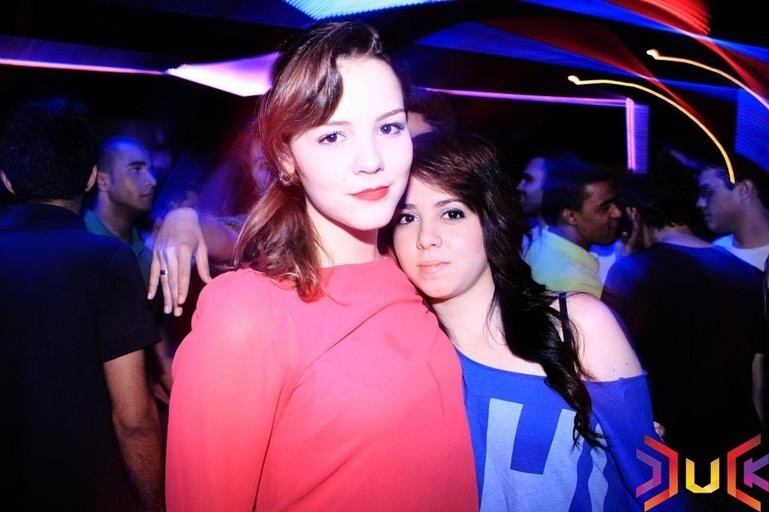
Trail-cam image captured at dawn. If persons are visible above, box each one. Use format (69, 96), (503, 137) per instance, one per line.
(1, 21), (768, 511)
